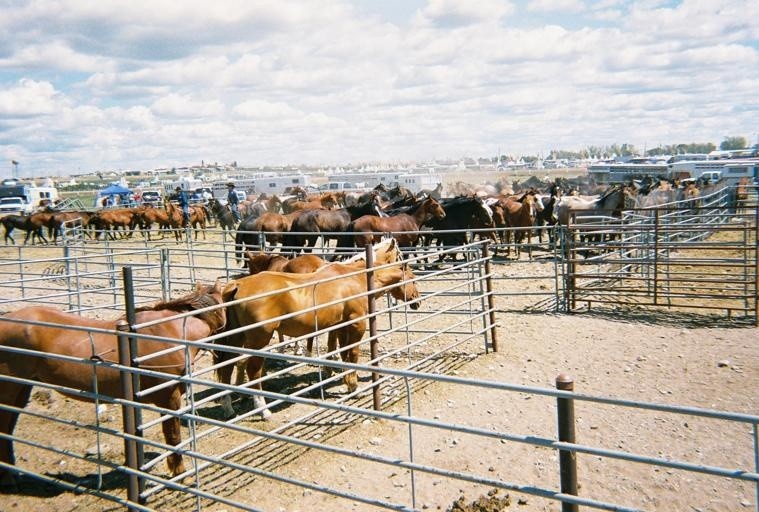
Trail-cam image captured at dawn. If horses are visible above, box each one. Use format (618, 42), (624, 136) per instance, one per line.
(235, 236), (405, 398)
(208, 180), (701, 271)
(212, 263), (422, 422)
(0, 203), (212, 245)
(0, 280), (226, 487)
(737, 177), (749, 213)
(246, 249), (329, 357)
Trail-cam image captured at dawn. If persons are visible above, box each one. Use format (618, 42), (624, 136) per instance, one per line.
(103, 192), (141, 208)
(202, 189), (212, 198)
(225, 182), (241, 223)
(172, 187), (191, 226)
(191, 191), (199, 203)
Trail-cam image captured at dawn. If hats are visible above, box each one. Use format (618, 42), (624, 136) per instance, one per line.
(227, 183), (235, 187)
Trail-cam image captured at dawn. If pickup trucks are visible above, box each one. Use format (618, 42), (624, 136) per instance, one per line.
(680, 171), (722, 184)
(140, 191), (163, 210)
(308, 181), (373, 194)
(0, 197), (35, 216)
